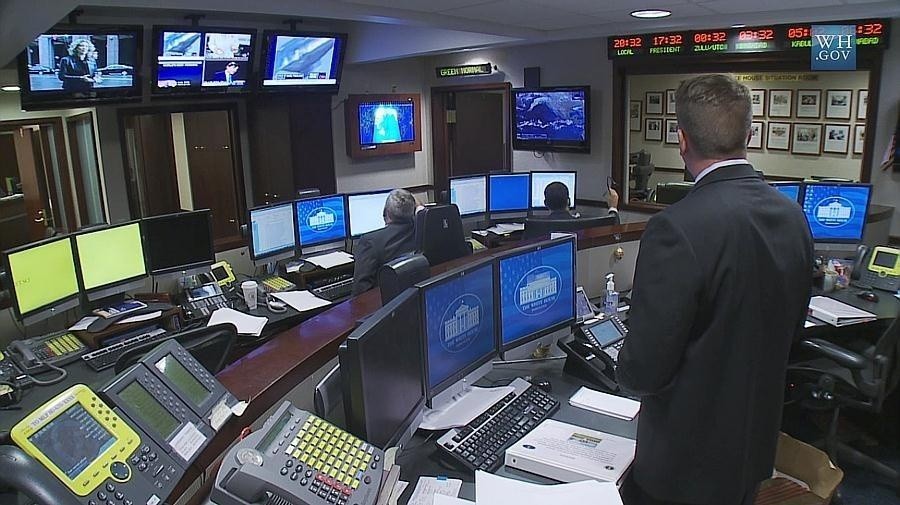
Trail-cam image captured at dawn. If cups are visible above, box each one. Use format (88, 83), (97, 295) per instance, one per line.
(241, 280), (259, 311)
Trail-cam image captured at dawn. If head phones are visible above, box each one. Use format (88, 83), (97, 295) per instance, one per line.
(606, 174), (622, 205)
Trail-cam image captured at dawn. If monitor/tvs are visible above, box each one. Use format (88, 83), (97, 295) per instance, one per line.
(491, 233), (577, 361)
(142, 209), (216, 280)
(448, 173), (488, 224)
(765, 180), (800, 209)
(857, 289), (880, 303)
(16, 22), (144, 112)
(355, 92), (422, 158)
(347, 188), (394, 246)
(259, 27), (349, 97)
(71, 219), (150, 301)
(509, 86), (591, 155)
(414, 255), (497, 409)
(488, 172), (530, 219)
(248, 201), (298, 267)
(530, 171), (577, 216)
(338, 287), (426, 462)
(148, 20), (258, 109)
(1, 233), (82, 327)
(802, 180), (874, 246)
(294, 193), (349, 255)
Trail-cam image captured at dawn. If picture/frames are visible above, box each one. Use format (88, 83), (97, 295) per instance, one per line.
(625, 81), (868, 160)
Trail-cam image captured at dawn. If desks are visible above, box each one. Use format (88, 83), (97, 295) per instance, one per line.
(2, 212), (599, 441)
(382, 257), (899, 503)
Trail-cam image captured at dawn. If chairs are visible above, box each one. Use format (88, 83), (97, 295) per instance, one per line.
(403, 201), (473, 267)
(518, 212), (621, 242)
(113, 322), (239, 380)
(783, 310), (898, 504)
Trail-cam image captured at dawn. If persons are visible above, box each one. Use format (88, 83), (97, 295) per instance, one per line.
(351, 189), (418, 298)
(541, 182), (619, 240)
(214, 62), (239, 82)
(616, 75), (815, 505)
(58, 39), (102, 90)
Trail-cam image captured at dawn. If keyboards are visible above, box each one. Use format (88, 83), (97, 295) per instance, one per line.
(435, 376), (561, 475)
(310, 273), (354, 301)
(81, 328), (173, 373)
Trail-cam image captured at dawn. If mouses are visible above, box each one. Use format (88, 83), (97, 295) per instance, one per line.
(527, 376), (552, 393)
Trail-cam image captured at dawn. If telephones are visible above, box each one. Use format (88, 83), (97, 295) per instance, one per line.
(556, 313), (629, 392)
(6, 329), (89, 375)
(210, 400), (384, 505)
(177, 282), (231, 321)
(235, 274), (296, 303)
(848, 244), (900, 294)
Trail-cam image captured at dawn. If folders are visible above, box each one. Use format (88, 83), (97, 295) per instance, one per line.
(807, 297), (877, 328)
(504, 418), (637, 490)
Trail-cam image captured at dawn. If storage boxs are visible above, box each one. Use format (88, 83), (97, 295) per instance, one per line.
(756, 430), (844, 503)
